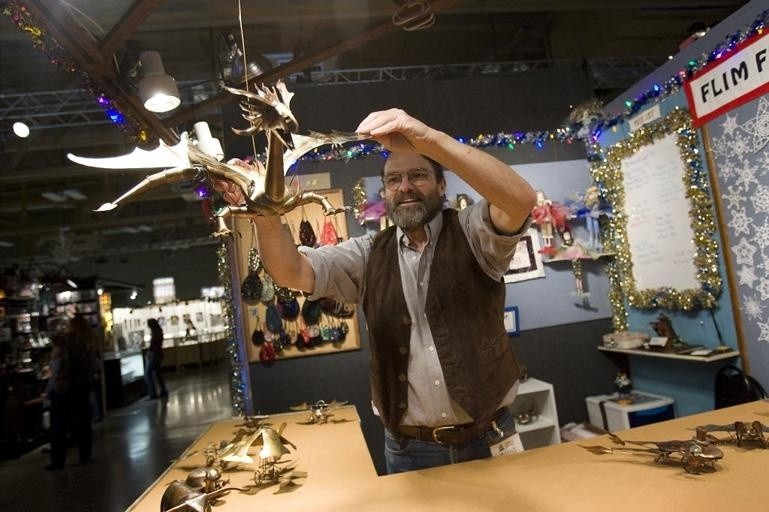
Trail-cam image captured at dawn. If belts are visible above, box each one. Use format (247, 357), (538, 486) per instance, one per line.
(389, 405), (510, 447)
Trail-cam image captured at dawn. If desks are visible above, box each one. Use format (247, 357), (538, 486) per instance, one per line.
(123, 400), (767, 511)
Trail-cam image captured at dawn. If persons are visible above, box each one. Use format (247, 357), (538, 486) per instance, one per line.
(210, 107), (539, 476)
(141, 317), (169, 400)
(41, 313), (103, 470)
(528, 185), (555, 249)
(580, 183), (605, 251)
(183, 319), (197, 340)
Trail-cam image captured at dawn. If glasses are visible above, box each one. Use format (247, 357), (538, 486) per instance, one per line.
(382, 167), (430, 192)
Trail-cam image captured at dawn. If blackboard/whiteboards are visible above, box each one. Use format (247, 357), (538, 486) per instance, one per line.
(617, 128), (703, 292)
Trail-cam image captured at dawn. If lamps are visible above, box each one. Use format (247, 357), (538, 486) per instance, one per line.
(125, 31), (275, 161)
(12, 118), (38, 139)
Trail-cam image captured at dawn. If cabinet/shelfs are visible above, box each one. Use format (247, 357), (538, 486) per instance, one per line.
(512, 377), (561, 450)
